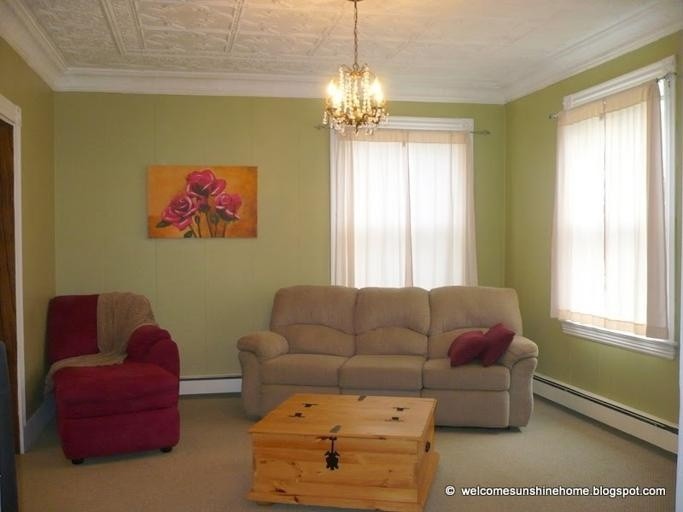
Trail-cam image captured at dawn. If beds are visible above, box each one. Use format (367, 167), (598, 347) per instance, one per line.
(245, 393), (437, 512)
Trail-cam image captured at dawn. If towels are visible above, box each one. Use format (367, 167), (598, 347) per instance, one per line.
(44, 294), (159, 391)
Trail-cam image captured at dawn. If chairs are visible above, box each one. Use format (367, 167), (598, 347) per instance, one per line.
(46, 294), (180, 466)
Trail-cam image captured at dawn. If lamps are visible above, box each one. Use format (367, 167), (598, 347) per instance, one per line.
(320, 0), (391, 137)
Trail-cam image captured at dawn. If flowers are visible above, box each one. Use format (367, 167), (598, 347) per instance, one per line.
(156, 170), (242, 238)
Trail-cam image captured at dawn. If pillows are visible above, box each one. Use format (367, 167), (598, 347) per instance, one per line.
(482, 323), (515, 367)
(447, 329), (489, 367)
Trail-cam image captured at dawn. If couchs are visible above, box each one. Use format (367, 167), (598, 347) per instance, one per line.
(237, 287), (538, 430)
